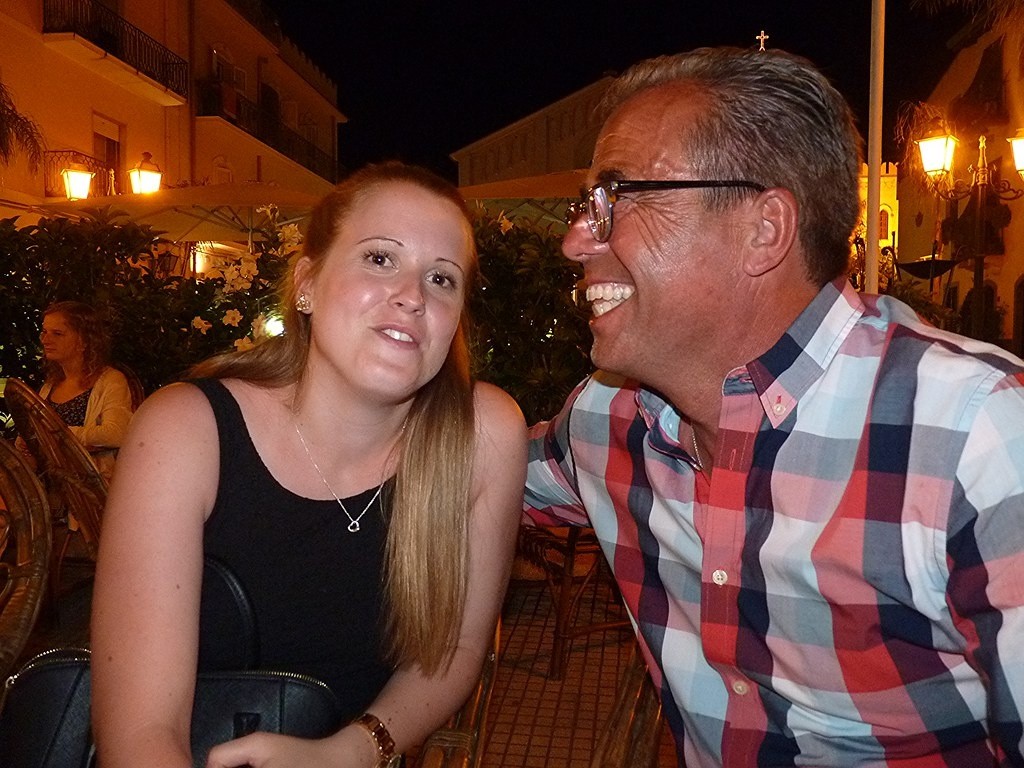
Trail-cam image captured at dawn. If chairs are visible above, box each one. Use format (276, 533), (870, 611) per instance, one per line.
(0, 379), (667, 768)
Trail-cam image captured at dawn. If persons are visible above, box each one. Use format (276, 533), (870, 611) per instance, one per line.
(87, 161), (527, 768)
(15, 299), (133, 546)
(520, 46), (1024, 768)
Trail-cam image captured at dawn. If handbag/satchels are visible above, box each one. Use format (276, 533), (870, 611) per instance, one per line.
(0, 646), (338, 768)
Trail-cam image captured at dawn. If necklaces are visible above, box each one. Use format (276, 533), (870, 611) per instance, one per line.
(291, 382), (409, 533)
(690, 422), (703, 471)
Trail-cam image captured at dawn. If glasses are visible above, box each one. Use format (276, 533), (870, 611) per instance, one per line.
(566, 177), (766, 243)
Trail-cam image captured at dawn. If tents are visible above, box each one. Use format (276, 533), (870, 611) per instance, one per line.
(26, 167), (591, 237)
